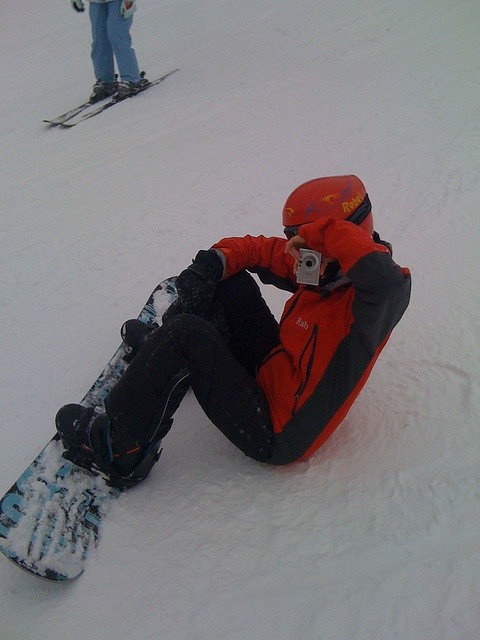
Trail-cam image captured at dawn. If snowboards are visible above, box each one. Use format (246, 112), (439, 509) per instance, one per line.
(1, 276), (192, 581)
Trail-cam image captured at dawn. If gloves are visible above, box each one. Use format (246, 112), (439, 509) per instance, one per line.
(119, 1), (138, 19)
(177, 249), (222, 303)
(72, 1), (86, 13)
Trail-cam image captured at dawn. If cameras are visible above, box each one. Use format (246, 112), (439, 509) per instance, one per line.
(294, 248), (321, 289)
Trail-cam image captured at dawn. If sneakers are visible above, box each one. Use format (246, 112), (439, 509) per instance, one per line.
(57, 404), (145, 476)
(119, 79), (138, 93)
(94, 79), (114, 92)
(120, 319), (154, 350)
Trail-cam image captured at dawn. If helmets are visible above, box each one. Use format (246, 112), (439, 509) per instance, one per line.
(281, 175), (374, 253)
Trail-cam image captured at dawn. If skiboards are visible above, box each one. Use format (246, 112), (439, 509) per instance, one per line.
(41, 67), (179, 128)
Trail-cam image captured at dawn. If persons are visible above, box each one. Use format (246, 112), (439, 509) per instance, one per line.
(69, 1), (149, 103)
(54, 174), (410, 490)
